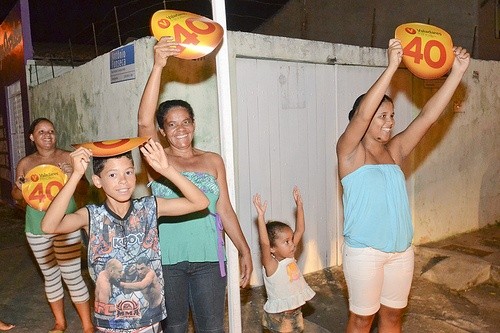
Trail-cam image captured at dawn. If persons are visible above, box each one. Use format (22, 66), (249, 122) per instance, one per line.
(0, 319), (16, 331)
(11, 118), (95, 333)
(95, 257), (122, 314)
(116, 257), (163, 325)
(336, 38), (470, 333)
(137, 35), (253, 333)
(251, 185), (316, 333)
(42, 139), (210, 333)
